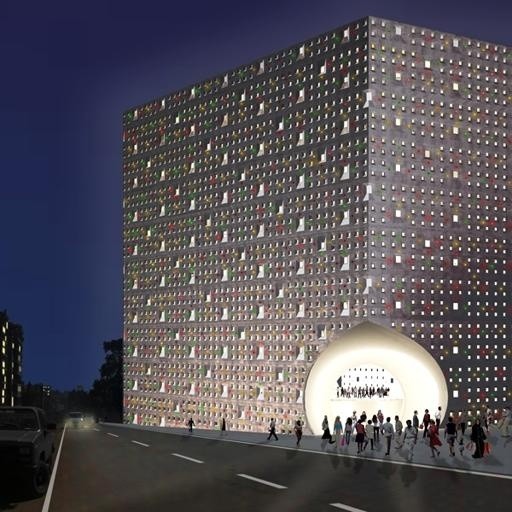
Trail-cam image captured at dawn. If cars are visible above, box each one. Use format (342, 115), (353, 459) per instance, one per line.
(0, 403), (58, 498)
(63, 410), (96, 430)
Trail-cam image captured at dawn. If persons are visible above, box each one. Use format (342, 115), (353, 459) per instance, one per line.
(320, 404), (512, 464)
(188, 417), (195, 433)
(336, 385), (390, 399)
(267, 418), (278, 441)
(294, 420), (304, 445)
(220, 418), (227, 436)
